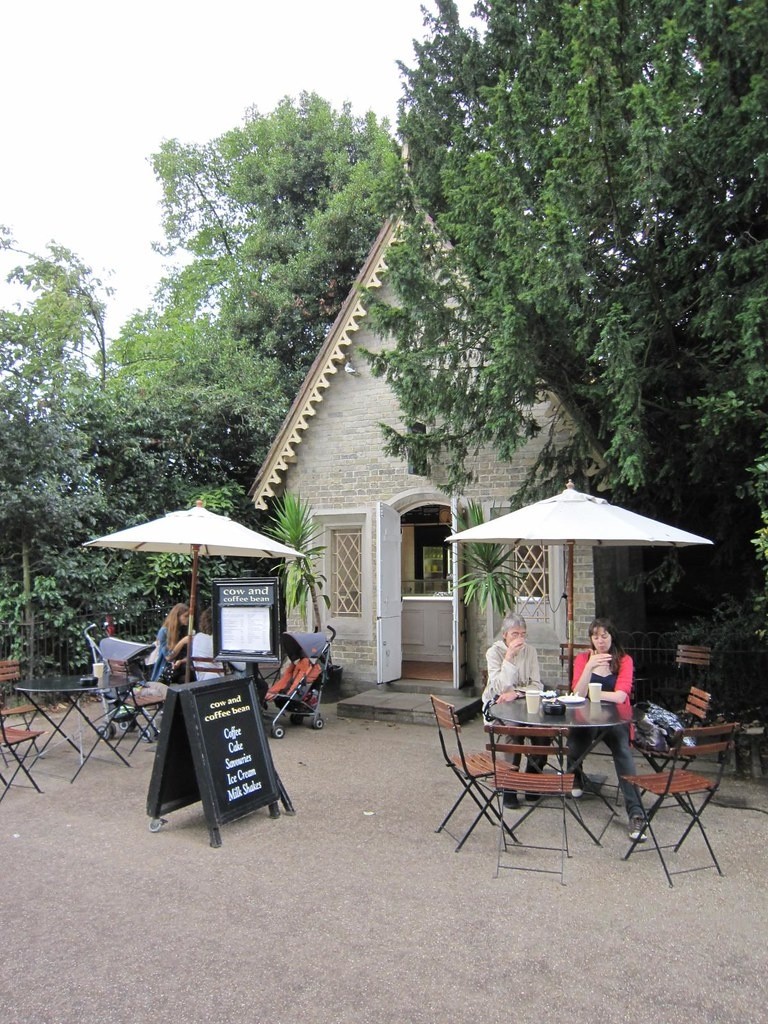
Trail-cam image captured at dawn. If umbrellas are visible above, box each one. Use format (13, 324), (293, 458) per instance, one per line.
(82, 500), (307, 683)
(444, 479), (714, 692)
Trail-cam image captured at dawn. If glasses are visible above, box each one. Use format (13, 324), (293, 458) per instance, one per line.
(507, 631), (528, 639)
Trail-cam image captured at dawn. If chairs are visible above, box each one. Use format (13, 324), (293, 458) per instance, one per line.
(430, 694), (523, 852)
(620, 722), (740, 888)
(653, 645), (712, 734)
(556, 643), (595, 699)
(108, 658), (166, 757)
(484, 724), (573, 887)
(0, 690), (45, 795)
(628, 685), (711, 814)
(0, 660), (46, 767)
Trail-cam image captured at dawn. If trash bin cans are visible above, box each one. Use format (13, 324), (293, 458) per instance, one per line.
(629, 678), (651, 705)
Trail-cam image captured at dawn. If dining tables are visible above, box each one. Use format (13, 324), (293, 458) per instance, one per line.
(491, 693), (645, 849)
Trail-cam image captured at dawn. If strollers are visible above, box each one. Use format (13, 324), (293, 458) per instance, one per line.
(259, 624), (336, 738)
(84, 621), (161, 740)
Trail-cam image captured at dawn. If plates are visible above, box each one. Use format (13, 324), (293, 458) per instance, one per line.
(557, 695), (586, 703)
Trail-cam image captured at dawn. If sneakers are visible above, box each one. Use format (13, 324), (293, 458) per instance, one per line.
(629, 816), (647, 843)
(571, 772), (584, 798)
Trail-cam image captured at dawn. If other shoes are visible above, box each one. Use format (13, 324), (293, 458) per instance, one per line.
(503, 789), (519, 808)
(524, 791), (540, 800)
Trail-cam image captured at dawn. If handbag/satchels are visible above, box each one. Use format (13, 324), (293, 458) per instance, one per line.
(643, 700), (695, 747)
(633, 713), (669, 752)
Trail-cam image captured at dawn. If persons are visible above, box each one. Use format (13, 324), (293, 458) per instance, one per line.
(151, 603), (195, 715)
(481, 612), (551, 810)
(192, 606), (245, 681)
(566, 618), (647, 841)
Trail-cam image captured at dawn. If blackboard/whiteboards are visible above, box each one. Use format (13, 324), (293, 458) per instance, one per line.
(145, 673), (278, 822)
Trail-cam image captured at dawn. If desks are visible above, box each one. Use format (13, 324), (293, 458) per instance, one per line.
(14, 675), (134, 772)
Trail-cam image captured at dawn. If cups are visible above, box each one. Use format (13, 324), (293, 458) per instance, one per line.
(92, 663), (104, 678)
(526, 691), (540, 714)
(588, 683), (602, 703)
